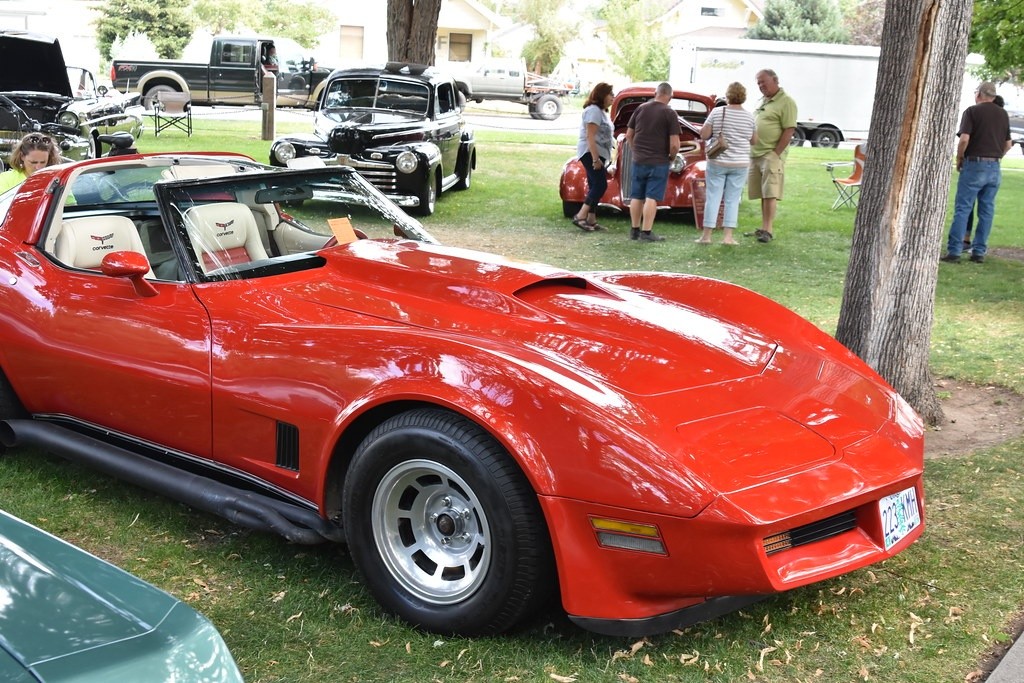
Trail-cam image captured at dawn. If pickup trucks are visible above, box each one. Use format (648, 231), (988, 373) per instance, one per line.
(110, 36), (331, 114)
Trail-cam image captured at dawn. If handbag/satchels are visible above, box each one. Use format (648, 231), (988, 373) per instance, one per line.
(705, 135), (729, 159)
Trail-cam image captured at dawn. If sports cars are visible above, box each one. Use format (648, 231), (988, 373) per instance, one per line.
(0, 150), (925, 637)
(559, 81), (728, 218)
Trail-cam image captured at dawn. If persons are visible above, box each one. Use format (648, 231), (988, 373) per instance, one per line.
(694, 82), (759, 246)
(626, 83), (682, 240)
(744, 69), (797, 242)
(1, 133), (77, 208)
(266, 45), (278, 65)
(571, 82), (615, 232)
(940, 81), (1012, 263)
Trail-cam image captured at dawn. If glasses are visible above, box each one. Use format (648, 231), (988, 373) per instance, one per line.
(974, 91), (981, 94)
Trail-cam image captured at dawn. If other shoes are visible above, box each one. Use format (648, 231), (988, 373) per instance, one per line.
(639, 231), (666, 241)
(940, 254), (961, 264)
(961, 240), (971, 250)
(630, 227), (640, 240)
(968, 254), (984, 263)
(757, 231), (772, 243)
(744, 228), (766, 237)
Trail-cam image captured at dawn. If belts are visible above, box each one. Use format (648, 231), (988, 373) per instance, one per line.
(970, 156), (997, 162)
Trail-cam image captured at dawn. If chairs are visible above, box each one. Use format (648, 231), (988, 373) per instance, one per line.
(154, 91), (193, 137)
(822, 143), (868, 211)
(184, 202), (266, 273)
(54, 214), (156, 280)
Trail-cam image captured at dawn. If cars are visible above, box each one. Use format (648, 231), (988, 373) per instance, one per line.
(267, 61), (477, 215)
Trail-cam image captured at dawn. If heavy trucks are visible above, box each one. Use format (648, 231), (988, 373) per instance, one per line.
(664, 35), (1024, 156)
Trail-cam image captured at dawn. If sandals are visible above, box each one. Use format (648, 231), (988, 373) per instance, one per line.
(585, 219), (603, 230)
(573, 214), (594, 231)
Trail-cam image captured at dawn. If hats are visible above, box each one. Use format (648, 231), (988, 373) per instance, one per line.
(976, 81), (996, 96)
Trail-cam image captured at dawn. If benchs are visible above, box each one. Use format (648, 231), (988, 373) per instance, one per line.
(344, 91), (426, 110)
